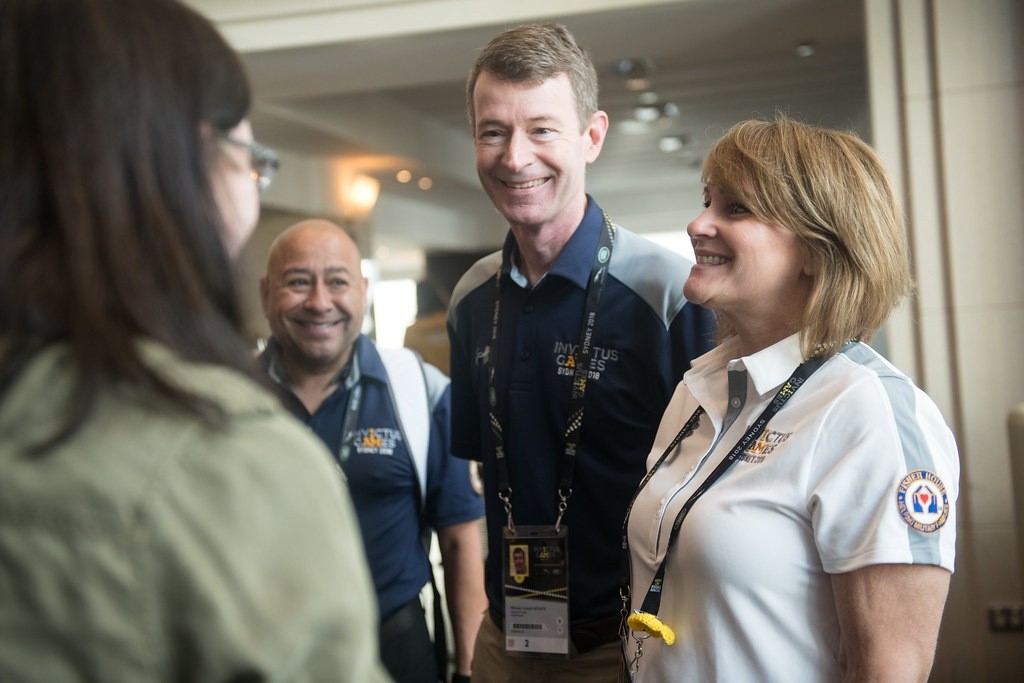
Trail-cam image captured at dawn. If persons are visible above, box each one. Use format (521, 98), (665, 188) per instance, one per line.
(0, 0), (394, 683)
(621, 117), (960, 683)
(512, 547), (527, 575)
(254, 219), (489, 683)
(446, 22), (720, 683)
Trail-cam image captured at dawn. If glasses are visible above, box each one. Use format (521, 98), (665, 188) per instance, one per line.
(205, 122), (282, 191)
